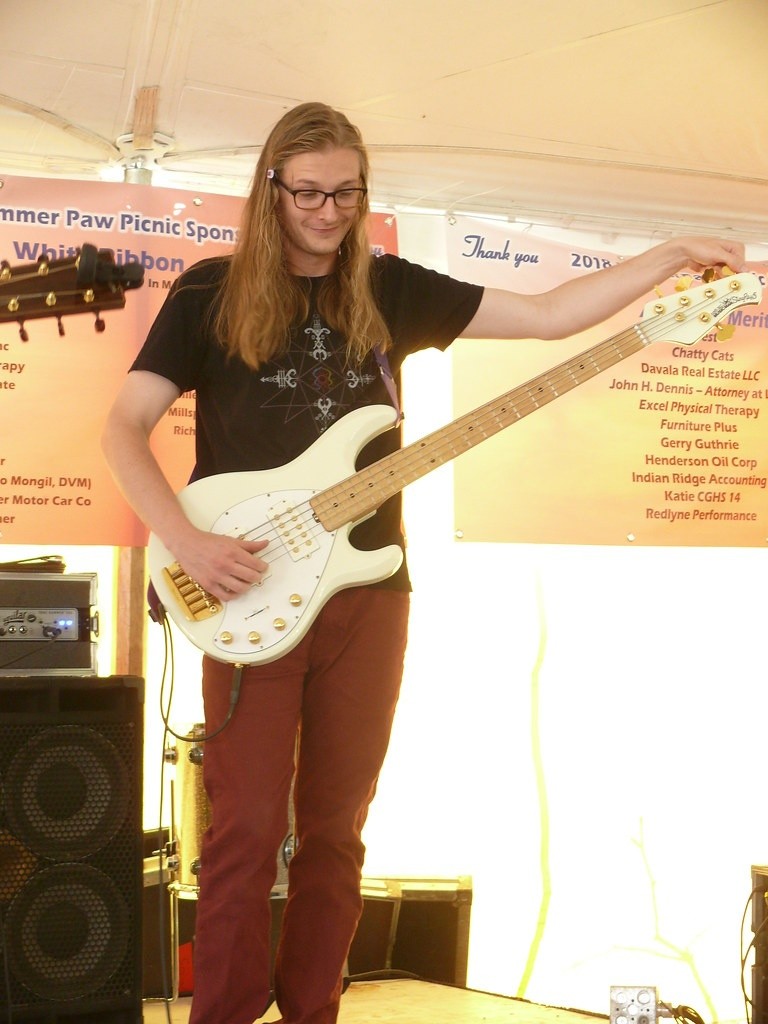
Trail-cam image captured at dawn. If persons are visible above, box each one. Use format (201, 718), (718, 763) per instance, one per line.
(93, 95), (751, 1024)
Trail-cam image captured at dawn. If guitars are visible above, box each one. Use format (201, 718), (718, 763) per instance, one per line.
(137, 265), (762, 673)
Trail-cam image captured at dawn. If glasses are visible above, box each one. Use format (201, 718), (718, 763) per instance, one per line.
(273, 175), (368, 209)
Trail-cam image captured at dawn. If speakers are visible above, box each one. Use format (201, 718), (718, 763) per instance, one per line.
(0, 674), (148, 1024)
